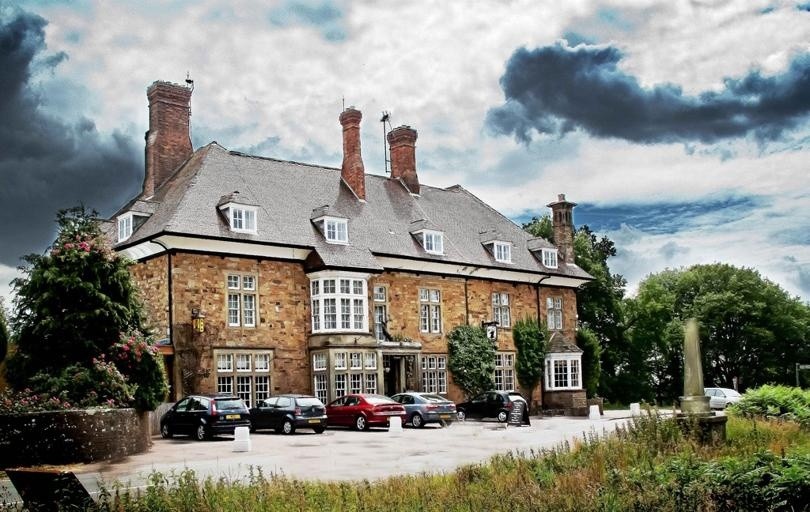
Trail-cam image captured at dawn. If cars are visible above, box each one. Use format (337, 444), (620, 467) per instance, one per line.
(390, 392), (458, 429)
(456, 391), (529, 423)
(703, 387), (744, 410)
(160, 395), (252, 440)
(248, 394), (328, 435)
(323, 394), (407, 430)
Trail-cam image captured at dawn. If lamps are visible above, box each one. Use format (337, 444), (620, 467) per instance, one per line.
(192, 308), (205, 334)
(482, 319), (499, 343)
